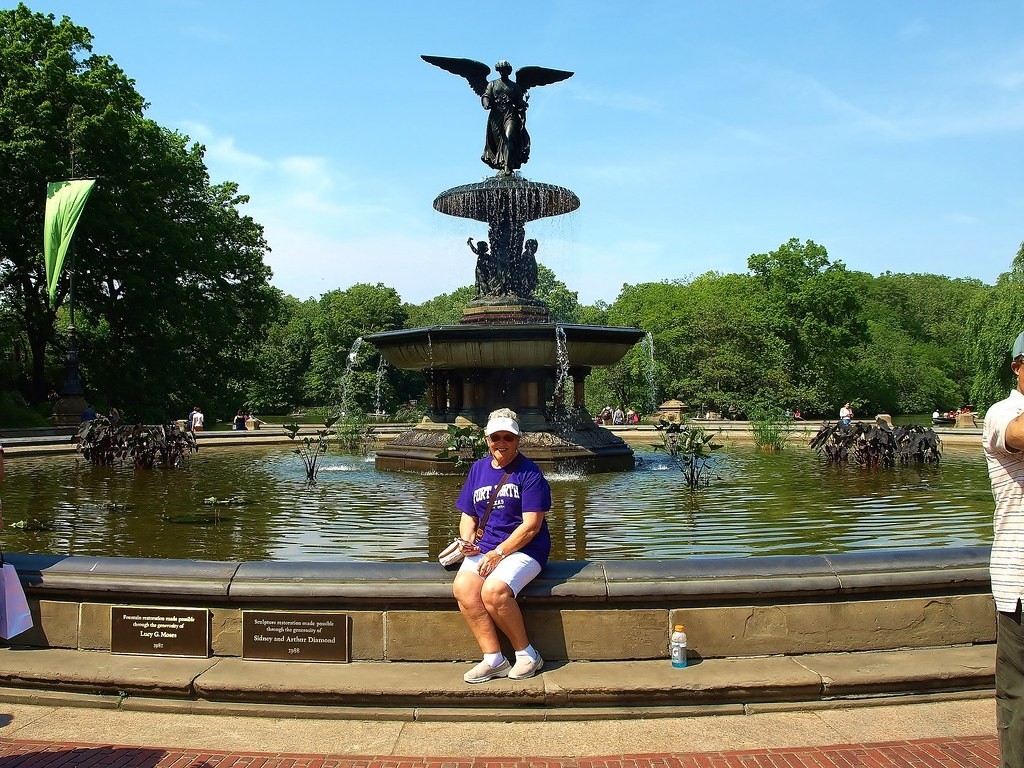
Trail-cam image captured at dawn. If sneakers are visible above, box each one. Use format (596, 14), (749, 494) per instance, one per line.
(508, 651), (544, 678)
(464, 655), (510, 683)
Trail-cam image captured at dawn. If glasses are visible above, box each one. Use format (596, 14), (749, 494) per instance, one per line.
(487, 434), (518, 442)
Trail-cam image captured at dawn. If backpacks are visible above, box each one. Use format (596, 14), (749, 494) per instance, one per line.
(615, 411), (622, 424)
(602, 408), (611, 420)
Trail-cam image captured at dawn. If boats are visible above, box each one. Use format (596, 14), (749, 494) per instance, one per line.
(931, 417), (956, 427)
(788, 416), (806, 421)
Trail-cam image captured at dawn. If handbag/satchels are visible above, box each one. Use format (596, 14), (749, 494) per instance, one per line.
(0, 551), (33, 639)
(439, 540), (467, 567)
(634, 413), (638, 423)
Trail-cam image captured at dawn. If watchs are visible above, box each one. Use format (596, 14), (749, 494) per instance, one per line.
(495, 548), (506, 559)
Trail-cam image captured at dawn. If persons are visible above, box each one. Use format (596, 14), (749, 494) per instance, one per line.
(189, 407), (204, 432)
(840, 401), (853, 425)
(932, 406), (969, 419)
(785, 408), (807, 421)
(47, 389), (58, 398)
(110, 406), (119, 428)
(453, 408), (551, 683)
(481, 60), (526, 176)
(522, 239), (538, 291)
(234, 407), (267, 429)
(595, 405), (636, 424)
(81, 404), (95, 421)
(982, 330), (1024, 768)
(467, 237), (492, 300)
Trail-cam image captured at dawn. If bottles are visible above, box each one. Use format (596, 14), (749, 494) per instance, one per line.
(672, 625), (687, 668)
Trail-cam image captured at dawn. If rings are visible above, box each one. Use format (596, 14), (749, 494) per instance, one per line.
(487, 566), (491, 571)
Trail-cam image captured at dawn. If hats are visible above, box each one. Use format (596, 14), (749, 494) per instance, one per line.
(1013, 331), (1024, 357)
(486, 418), (519, 435)
